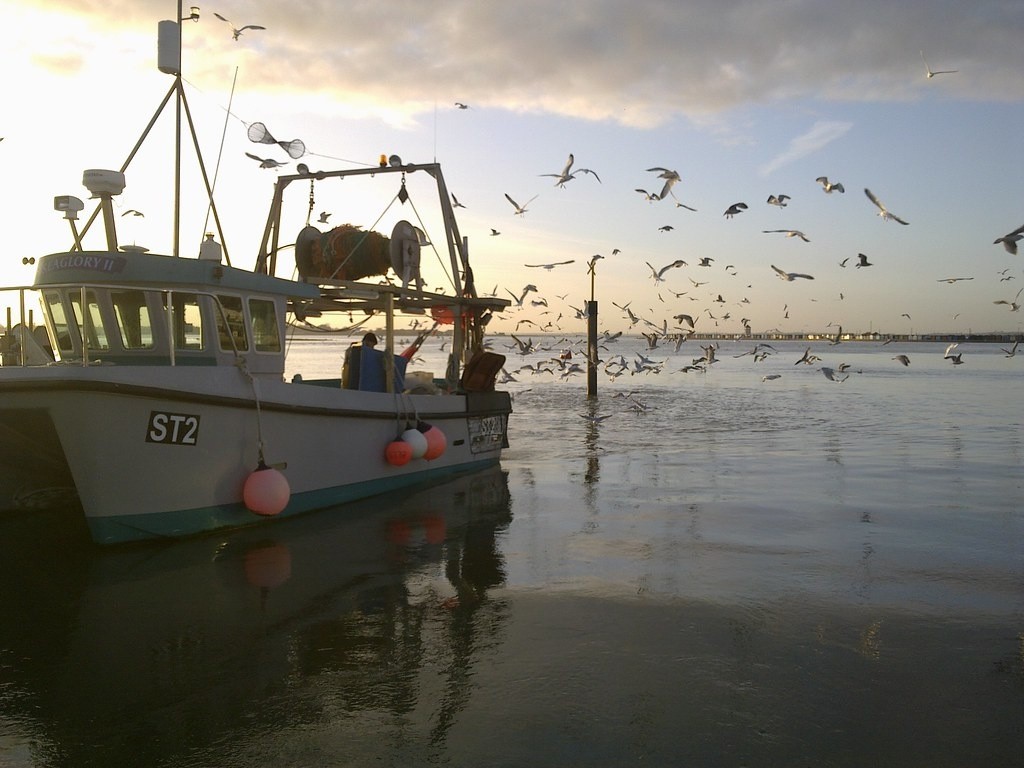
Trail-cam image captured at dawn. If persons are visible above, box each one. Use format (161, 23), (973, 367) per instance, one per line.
(341, 332), (378, 389)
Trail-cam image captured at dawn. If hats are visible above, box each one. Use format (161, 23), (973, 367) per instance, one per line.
(362, 333), (378, 344)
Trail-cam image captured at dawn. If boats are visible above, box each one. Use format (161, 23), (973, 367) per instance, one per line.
(0, 0), (517, 545)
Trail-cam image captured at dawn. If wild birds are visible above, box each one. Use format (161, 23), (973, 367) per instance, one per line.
(212, 12), (266, 41)
(453, 102), (471, 109)
(316, 212), (332, 224)
(120, 210), (144, 217)
(378, 153), (1024, 426)
(919, 49), (959, 78)
(244, 152), (288, 169)
